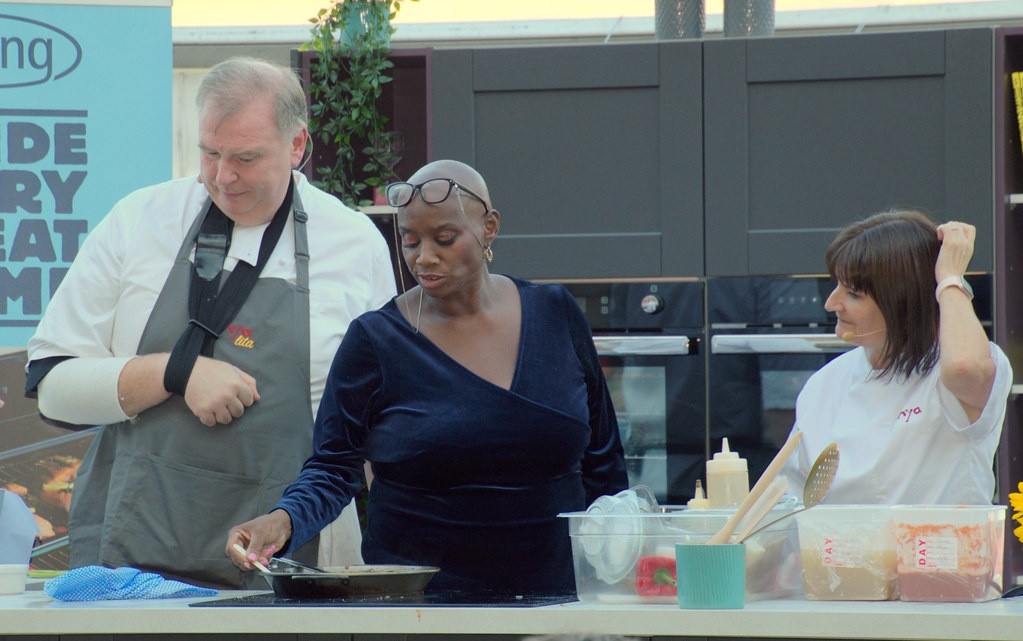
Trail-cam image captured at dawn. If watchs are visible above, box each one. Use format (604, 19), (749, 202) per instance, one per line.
(936, 276), (975, 303)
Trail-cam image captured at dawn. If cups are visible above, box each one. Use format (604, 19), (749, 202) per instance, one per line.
(676, 542), (747, 609)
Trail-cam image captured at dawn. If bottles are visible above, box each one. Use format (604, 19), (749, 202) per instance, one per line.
(688, 480), (708, 509)
(706, 437), (750, 510)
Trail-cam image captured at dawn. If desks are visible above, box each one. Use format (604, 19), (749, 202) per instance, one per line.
(1, 562), (1023, 641)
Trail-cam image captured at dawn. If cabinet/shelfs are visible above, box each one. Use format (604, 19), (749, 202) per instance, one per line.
(301, 25), (1023, 594)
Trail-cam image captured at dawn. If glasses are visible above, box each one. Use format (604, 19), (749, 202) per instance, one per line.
(385, 178), (489, 215)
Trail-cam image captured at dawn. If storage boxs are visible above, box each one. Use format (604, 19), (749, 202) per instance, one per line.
(557, 496), (799, 603)
(800, 504), (888, 599)
(889, 503), (1008, 603)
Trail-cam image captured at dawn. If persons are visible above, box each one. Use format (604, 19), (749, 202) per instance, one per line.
(223, 159), (629, 596)
(791, 209), (1013, 507)
(22, 58), (401, 591)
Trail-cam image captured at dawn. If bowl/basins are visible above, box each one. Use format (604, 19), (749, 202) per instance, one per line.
(259, 565), (440, 597)
(0, 564), (28, 596)
